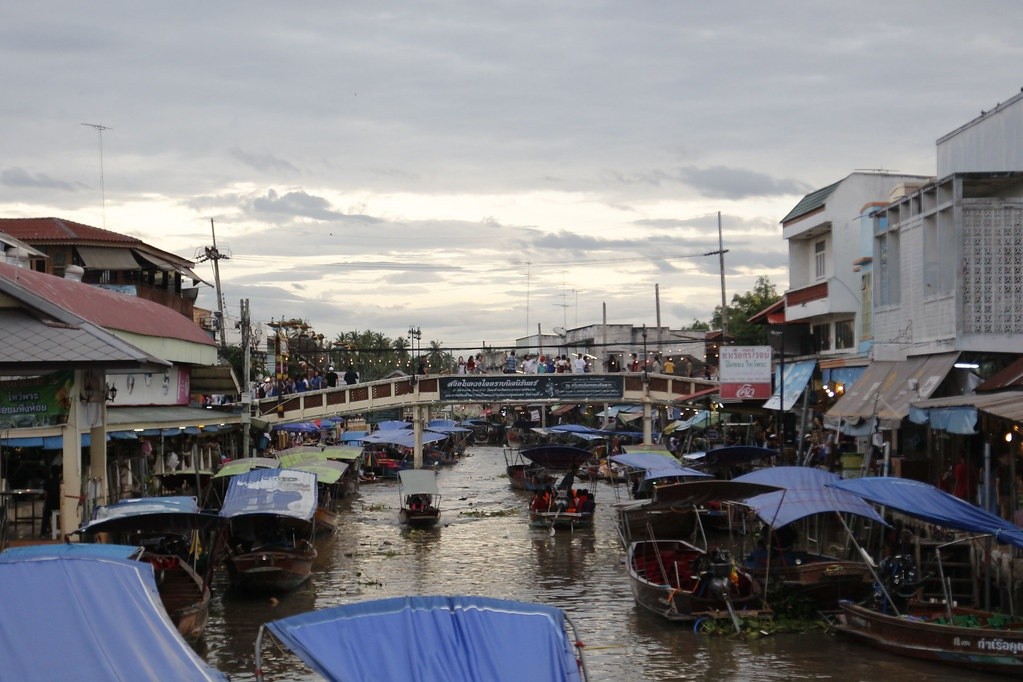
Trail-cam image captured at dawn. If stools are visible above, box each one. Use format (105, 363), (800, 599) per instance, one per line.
(49, 508), (60, 540)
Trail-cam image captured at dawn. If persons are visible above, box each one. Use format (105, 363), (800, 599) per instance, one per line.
(685, 358), (692, 378)
(763, 423), (774, 448)
(257, 367), (322, 399)
(702, 365), (711, 381)
(823, 428), (835, 466)
(466, 353), (487, 374)
(757, 522), (798, 551)
(406, 494), (432, 511)
(439, 368), (450, 374)
(530, 486), (596, 514)
(951, 449), (1012, 520)
(503, 351), (675, 375)
(417, 355), (428, 378)
(38, 465), (64, 539)
(343, 366), (359, 386)
(324, 366), (339, 388)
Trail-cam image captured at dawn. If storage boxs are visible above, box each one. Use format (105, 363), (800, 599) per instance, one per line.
(839, 434), (878, 479)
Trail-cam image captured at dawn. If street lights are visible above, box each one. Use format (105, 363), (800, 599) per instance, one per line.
(298, 334), (309, 360)
(408, 326), (422, 383)
(642, 325), (648, 382)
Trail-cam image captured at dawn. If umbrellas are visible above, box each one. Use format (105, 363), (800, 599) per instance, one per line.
(282, 416), (343, 433)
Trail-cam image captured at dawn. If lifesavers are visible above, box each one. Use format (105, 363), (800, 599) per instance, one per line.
(693, 617), (708, 633)
(398, 508), (408, 524)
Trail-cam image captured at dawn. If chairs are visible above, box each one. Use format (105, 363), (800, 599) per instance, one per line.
(12, 493), (37, 539)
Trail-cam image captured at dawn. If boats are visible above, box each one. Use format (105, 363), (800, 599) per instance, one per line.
(63, 415), (472, 649)
(393, 468), (442, 526)
(623, 480), (787, 625)
(532, 422), (780, 532)
(501, 426), (557, 489)
(727, 468), (1023, 666)
(520, 447), (601, 527)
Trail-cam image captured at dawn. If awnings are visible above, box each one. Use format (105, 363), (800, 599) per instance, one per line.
(822, 352), (962, 435)
(761, 359), (819, 411)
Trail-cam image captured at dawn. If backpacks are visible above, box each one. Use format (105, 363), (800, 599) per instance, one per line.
(258, 384), (266, 398)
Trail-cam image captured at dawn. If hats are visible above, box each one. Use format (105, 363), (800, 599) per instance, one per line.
(288, 378), (294, 383)
(264, 377), (271, 382)
(329, 367), (333, 370)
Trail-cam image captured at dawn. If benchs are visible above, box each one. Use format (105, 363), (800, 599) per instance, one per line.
(634, 545), (700, 585)
